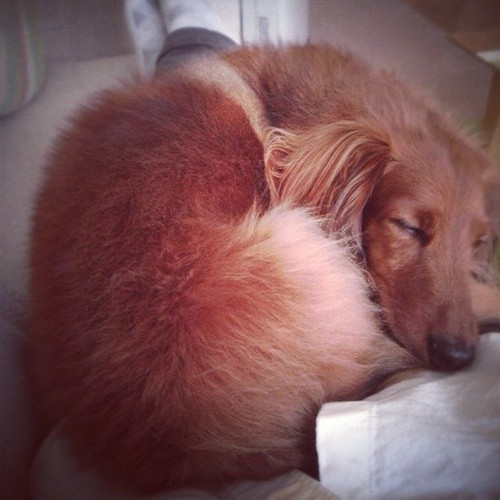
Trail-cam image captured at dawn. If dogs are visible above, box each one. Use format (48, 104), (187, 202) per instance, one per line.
(26, 33), (500, 494)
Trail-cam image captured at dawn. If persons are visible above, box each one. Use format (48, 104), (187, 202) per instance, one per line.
(0, 1), (500, 500)
